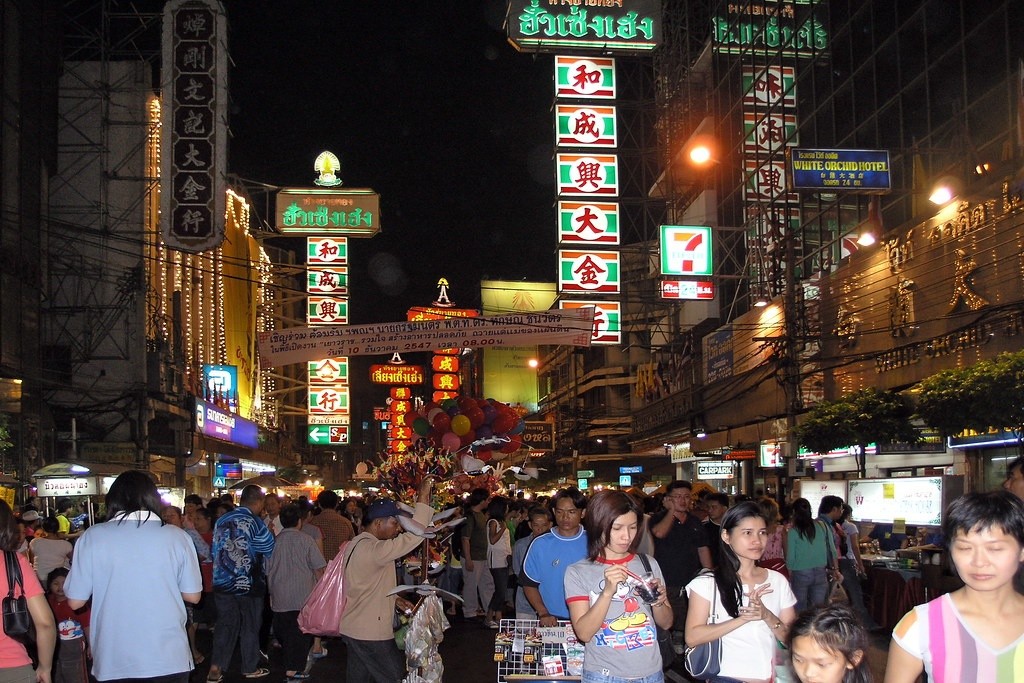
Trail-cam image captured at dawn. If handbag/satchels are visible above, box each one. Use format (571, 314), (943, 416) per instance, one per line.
(506, 562), (518, 588)
(295, 540), (352, 636)
(249, 567), (267, 597)
(828, 581), (848, 606)
(2, 551), (36, 647)
(637, 553), (677, 670)
(684, 571), (722, 681)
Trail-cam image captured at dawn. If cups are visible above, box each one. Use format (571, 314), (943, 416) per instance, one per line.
(884, 559), (901, 570)
(921, 551), (942, 565)
(630, 571), (661, 603)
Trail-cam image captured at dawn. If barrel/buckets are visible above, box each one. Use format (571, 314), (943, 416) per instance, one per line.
(895, 548), (923, 570)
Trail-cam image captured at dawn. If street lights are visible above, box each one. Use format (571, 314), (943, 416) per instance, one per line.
(690, 143), (800, 509)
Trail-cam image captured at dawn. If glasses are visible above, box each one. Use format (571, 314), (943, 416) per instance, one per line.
(669, 495), (690, 499)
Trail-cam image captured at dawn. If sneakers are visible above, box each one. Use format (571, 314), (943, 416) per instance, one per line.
(484, 618), (514, 628)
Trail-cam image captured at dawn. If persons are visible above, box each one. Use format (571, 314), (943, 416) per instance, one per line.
(0, 453), (1024, 683)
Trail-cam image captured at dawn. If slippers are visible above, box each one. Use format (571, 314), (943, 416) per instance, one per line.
(312, 646), (328, 658)
(286, 670), (311, 679)
(194, 654), (207, 666)
(246, 668), (270, 678)
(206, 674), (223, 683)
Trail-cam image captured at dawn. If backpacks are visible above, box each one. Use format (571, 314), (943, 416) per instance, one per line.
(451, 512), (477, 560)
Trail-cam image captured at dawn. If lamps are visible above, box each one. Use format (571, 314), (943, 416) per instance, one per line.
(928, 176), (963, 204)
(856, 229), (882, 249)
(754, 297), (772, 308)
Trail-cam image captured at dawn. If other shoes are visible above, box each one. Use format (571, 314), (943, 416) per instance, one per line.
(477, 609), (486, 615)
(672, 632), (684, 654)
(447, 610), (456, 615)
(863, 624), (886, 631)
(465, 617), (482, 623)
(272, 643), (283, 650)
(258, 650), (269, 663)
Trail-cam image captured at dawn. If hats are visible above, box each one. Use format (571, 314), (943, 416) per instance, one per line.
(22, 510), (43, 521)
(366, 499), (398, 520)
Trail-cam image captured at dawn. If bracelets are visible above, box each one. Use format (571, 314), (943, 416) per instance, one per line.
(831, 567), (839, 570)
(655, 602), (664, 607)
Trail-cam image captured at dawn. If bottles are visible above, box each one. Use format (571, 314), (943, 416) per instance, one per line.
(78, 521), (84, 534)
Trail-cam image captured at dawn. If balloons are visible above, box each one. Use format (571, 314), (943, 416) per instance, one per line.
(403, 395), (524, 482)
(356, 462), (367, 477)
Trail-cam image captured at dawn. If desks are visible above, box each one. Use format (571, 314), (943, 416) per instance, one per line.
(840, 554), (900, 622)
(882, 564), (947, 633)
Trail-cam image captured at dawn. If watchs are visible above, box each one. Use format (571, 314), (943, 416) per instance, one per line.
(771, 620), (783, 631)
